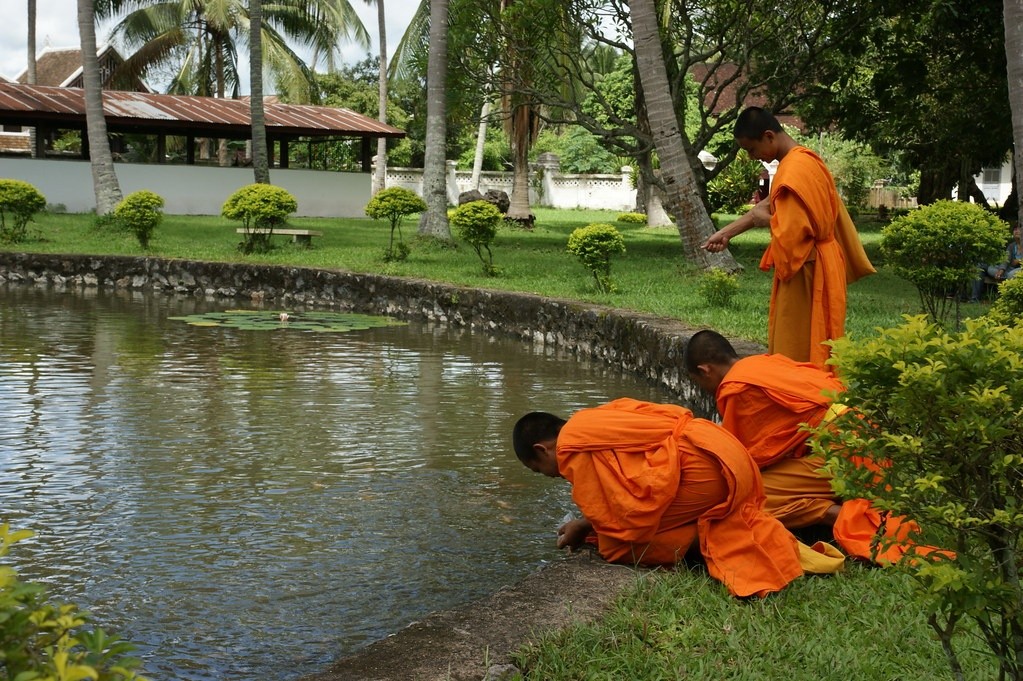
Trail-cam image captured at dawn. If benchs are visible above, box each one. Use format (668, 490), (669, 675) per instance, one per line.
(235, 227), (323, 243)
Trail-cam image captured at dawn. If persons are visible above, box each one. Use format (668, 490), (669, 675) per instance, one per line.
(513, 398), (847, 599)
(700, 106), (878, 379)
(686, 329), (958, 568)
(971, 223), (1023, 304)
